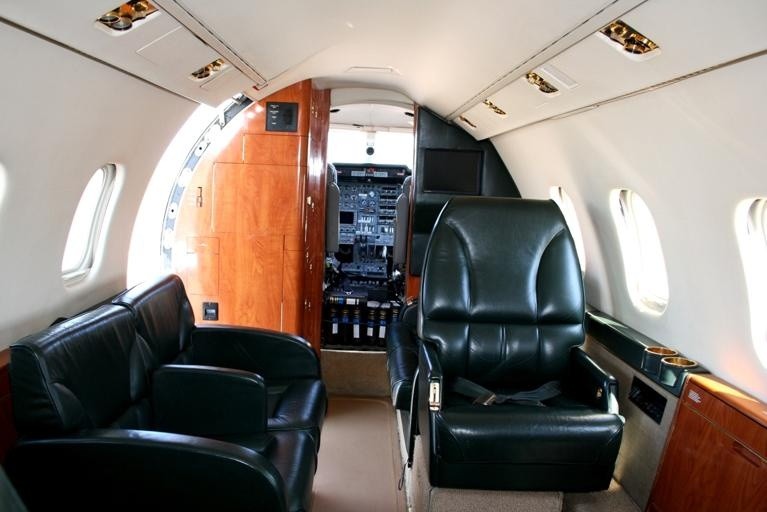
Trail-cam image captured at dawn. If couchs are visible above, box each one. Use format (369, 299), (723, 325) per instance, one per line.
(386, 298), (419, 410)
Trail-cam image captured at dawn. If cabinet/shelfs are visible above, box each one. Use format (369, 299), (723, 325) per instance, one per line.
(645, 373), (767, 512)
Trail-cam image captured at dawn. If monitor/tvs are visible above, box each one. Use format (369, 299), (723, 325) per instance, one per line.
(423, 148), (483, 195)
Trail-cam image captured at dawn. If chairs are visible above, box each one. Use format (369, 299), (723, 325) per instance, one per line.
(7, 304), (318, 512)
(415, 194), (626, 493)
(111, 273), (328, 458)
